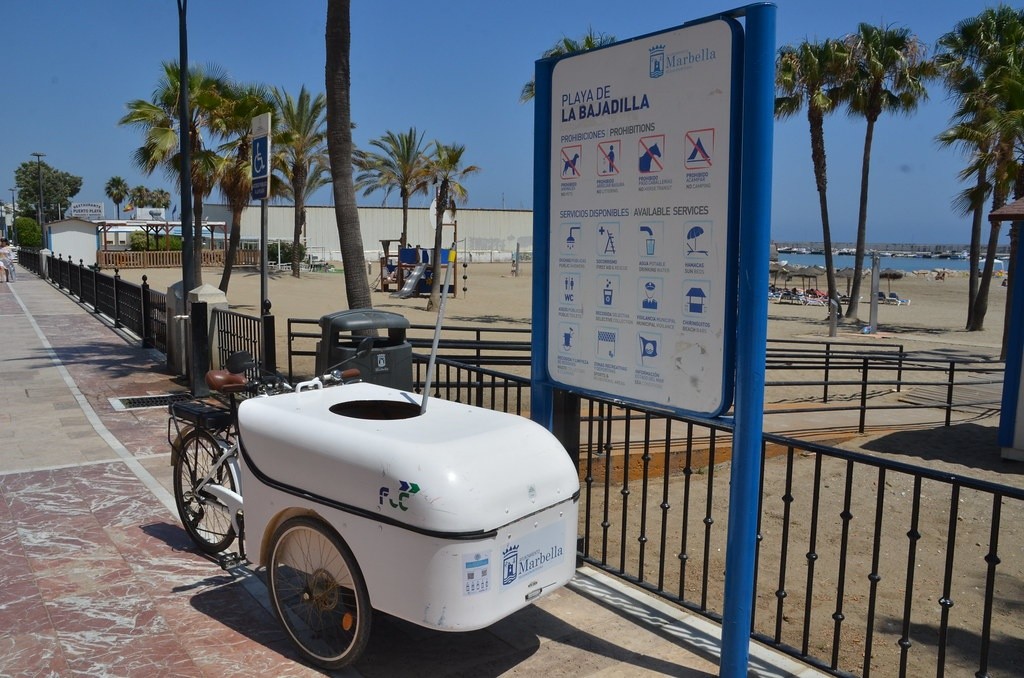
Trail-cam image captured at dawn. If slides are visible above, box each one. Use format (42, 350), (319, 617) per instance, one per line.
(389, 263), (429, 300)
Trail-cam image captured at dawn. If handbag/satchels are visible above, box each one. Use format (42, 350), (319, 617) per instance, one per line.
(10, 265), (16, 280)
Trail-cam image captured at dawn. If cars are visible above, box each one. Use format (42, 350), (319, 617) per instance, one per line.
(11, 248), (18, 259)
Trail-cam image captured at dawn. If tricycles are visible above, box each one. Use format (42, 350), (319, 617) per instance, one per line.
(167, 369), (581, 671)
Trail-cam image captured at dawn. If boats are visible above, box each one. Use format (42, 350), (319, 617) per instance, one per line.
(796, 248), (806, 254)
(777, 247), (797, 253)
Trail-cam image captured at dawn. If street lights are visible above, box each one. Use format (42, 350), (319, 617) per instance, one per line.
(8, 187), (18, 246)
(29, 151), (48, 249)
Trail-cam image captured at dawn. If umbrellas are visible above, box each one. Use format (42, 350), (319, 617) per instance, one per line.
(834, 266), (855, 298)
(879, 267), (903, 294)
(769, 263), (826, 295)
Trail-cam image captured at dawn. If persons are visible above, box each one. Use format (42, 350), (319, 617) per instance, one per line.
(511, 260), (516, 277)
(404, 268), (412, 279)
(0, 237), (15, 283)
(387, 267), (398, 283)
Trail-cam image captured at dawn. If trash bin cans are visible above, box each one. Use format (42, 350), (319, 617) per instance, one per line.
(314, 308), (413, 393)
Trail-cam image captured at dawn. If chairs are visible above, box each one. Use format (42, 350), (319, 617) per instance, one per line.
(768, 286), (910, 308)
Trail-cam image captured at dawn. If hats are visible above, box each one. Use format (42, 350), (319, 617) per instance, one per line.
(0, 237), (7, 242)
